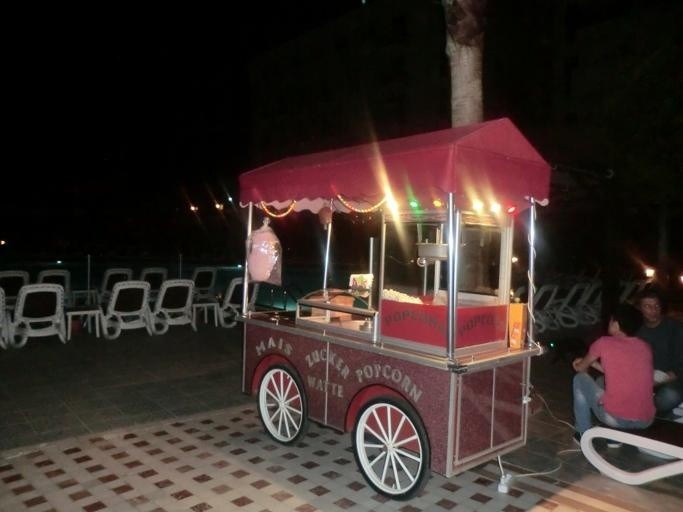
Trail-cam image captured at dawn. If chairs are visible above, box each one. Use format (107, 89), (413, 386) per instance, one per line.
(560, 283), (591, 329)
(0, 289), (13, 349)
(105, 280), (153, 339)
(37, 269), (70, 297)
(155, 279), (199, 331)
(97, 269), (133, 312)
(0, 270), (30, 310)
(190, 266), (217, 298)
(140, 268), (168, 301)
(513, 285), (536, 301)
(12, 283), (68, 349)
(591, 281), (604, 325)
(219, 277), (260, 328)
(581, 417), (682, 486)
(534, 285), (559, 334)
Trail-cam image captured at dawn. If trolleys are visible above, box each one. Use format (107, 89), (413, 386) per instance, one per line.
(237, 118), (552, 502)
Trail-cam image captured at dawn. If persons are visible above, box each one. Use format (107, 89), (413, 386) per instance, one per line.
(635, 292), (683, 411)
(573, 304), (656, 449)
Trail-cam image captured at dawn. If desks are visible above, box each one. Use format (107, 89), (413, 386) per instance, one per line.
(67, 303), (104, 339)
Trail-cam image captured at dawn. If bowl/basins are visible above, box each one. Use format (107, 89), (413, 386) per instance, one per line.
(414, 242), (448, 261)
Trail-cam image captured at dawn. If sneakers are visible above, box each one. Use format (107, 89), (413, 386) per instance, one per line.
(573, 432), (583, 444)
(607, 437), (622, 448)
